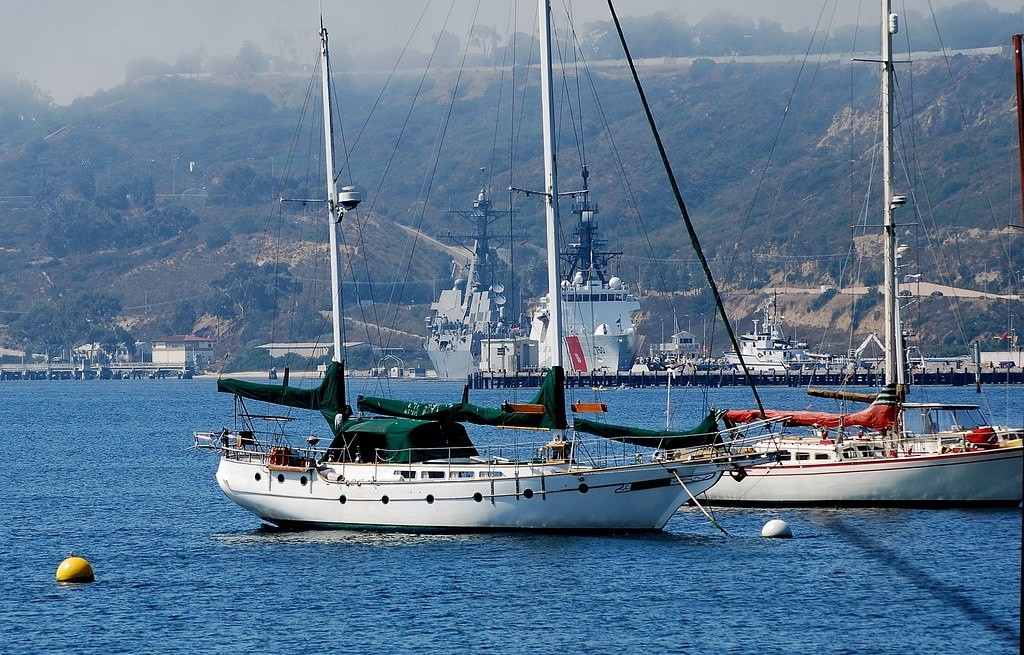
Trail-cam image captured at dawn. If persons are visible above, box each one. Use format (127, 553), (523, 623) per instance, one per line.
(945, 359), (950, 365)
(788, 363), (803, 370)
(821, 429), (829, 439)
(816, 344), (820, 354)
(999, 331), (1008, 348)
(906, 363), (923, 374)
(808, 363), (847, 369)
(959, 361), (963, 366)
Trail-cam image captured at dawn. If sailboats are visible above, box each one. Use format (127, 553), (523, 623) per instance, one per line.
(183, 0), (1024, 538)
